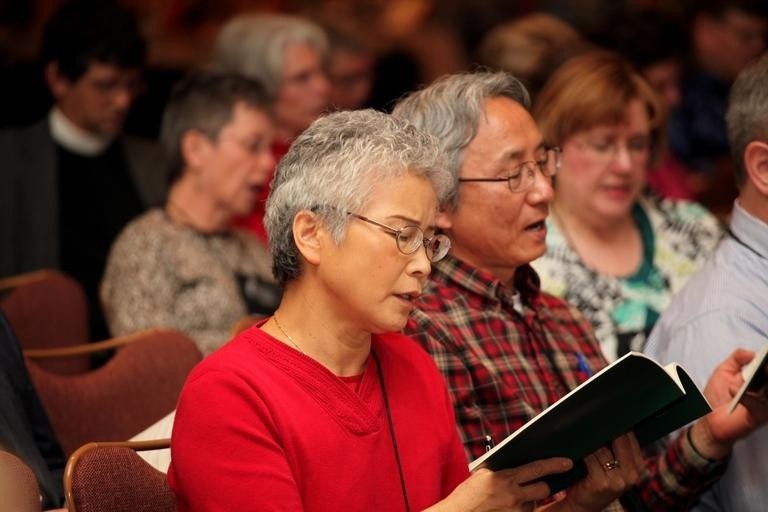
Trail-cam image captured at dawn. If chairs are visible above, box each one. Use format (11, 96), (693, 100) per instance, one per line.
(22, 329), (203, 458)
(62, 439), (176, 512)
(0, 448), (43, 512)
(0, 267), (89, 377)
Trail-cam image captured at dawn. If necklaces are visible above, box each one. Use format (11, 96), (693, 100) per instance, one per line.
(271, 309), (303, 354)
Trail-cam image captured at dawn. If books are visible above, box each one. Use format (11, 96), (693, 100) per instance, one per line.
(466, 349), (712, 485)
(728, 346), (766, 416)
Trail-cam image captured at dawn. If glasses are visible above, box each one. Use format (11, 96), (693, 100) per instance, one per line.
(311, 205), (452, 263)
(458, 146), (563, 193)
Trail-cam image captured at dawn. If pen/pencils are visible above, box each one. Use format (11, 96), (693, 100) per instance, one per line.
(577, 352), (593, 379)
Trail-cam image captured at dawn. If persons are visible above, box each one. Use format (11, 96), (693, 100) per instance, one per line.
(391, 72), (767, 511)
(641, 54), (766, 512)
(0, 0), (768, 366)
(166, 108), (647, 512)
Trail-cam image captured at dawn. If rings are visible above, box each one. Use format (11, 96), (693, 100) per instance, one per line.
(603, 460), (620, 471)
